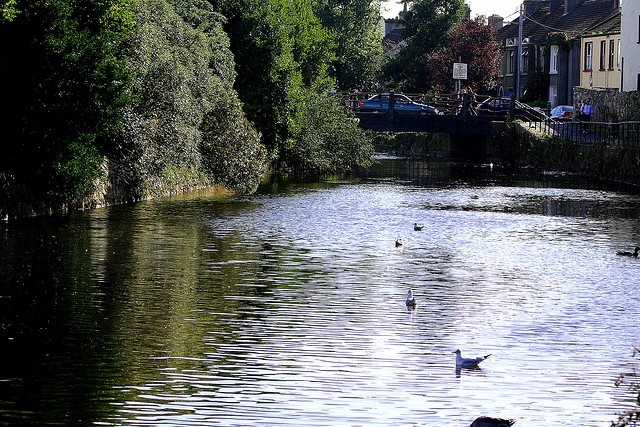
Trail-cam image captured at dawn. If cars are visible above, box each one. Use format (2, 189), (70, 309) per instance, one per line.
(550, 105), (574, 119)
(477, 97), (545, 120)
(360, 92), (436, 114)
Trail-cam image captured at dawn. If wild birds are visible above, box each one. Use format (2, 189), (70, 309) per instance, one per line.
(395, 239), (402, 247)
(412, 223), (424, 231)
(406, 290), (417, 306)
(452, 349), (492, 367)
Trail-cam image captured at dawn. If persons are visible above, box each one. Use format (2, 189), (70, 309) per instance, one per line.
(588, 75), (593, 87)
(461, 89), (467, 93)
(573, 98), (593, 122)
(466, 87), (473, 93)
